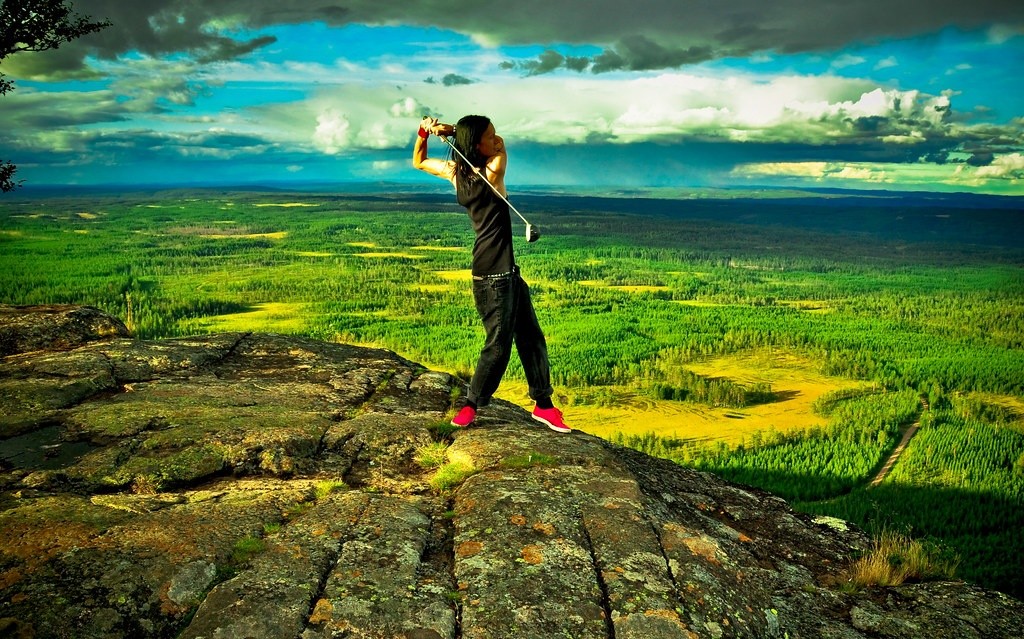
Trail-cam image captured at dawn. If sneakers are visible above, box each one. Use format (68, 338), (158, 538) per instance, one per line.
(531, 405), (571, 433)
(450, 406), (476, 427)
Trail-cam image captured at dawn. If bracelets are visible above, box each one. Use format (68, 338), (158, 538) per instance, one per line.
(418, 127), (429, 138)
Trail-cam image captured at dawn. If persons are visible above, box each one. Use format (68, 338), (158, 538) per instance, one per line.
(411, 114), (574, 436)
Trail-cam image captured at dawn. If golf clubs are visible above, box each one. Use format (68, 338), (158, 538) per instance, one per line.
(423, 114), (541, 242)
